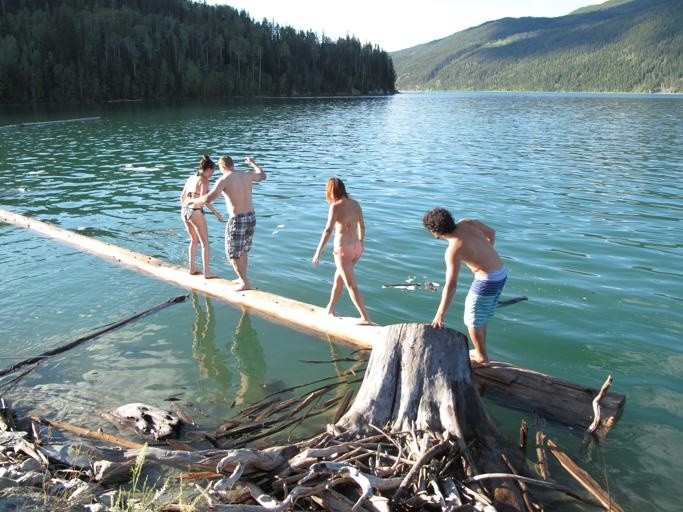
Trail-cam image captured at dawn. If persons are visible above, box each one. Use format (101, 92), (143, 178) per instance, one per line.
(189, 294), (232, 391)
(311, 178), (373, 326)
(180, 154), (224, 278)
(425, 206), (507, 367)
(231, 305), (272, 418)
(192, 155), (267, 291)
